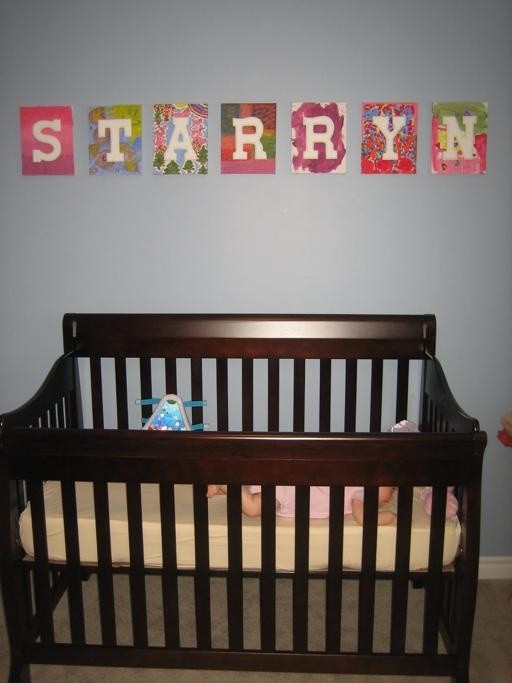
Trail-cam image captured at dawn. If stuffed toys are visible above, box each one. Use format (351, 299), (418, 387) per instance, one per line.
(134, 393), (210, 431)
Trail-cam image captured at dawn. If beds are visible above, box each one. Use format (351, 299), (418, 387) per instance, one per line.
(0, 313), (488, 683)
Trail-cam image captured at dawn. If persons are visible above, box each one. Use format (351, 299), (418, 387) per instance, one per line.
(204, 483), (399, 527)
